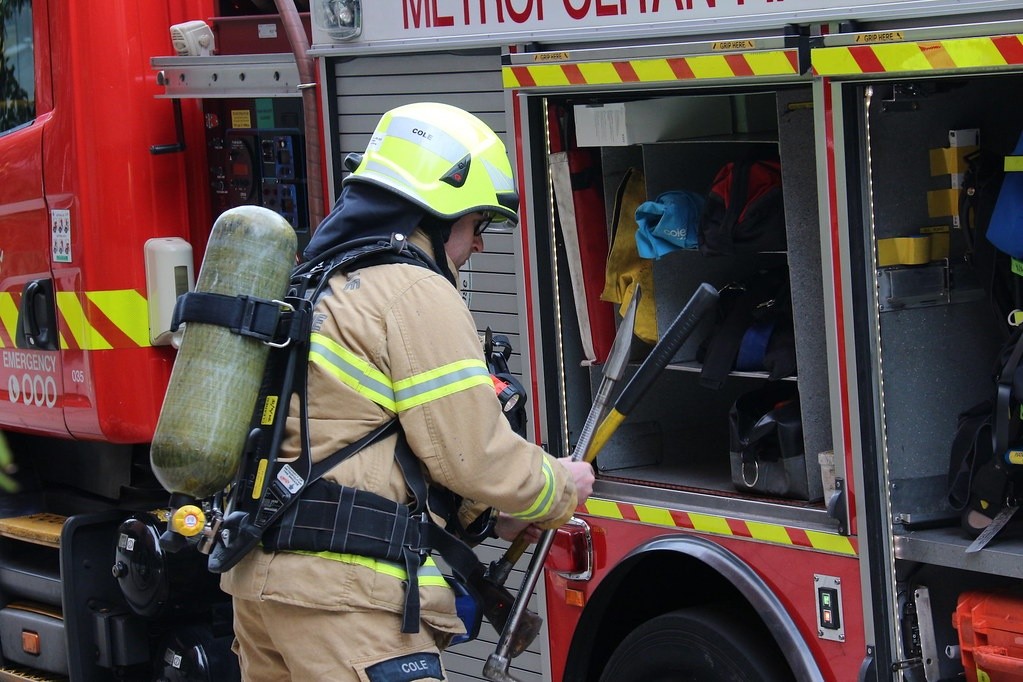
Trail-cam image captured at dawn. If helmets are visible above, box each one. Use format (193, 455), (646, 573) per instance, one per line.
(340, 102), (520, 230)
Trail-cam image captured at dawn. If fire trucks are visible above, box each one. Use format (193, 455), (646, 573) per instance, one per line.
(2, 1), (1022, 680)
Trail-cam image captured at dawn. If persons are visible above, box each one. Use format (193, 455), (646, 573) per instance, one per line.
(221, 100), (595, 678)
